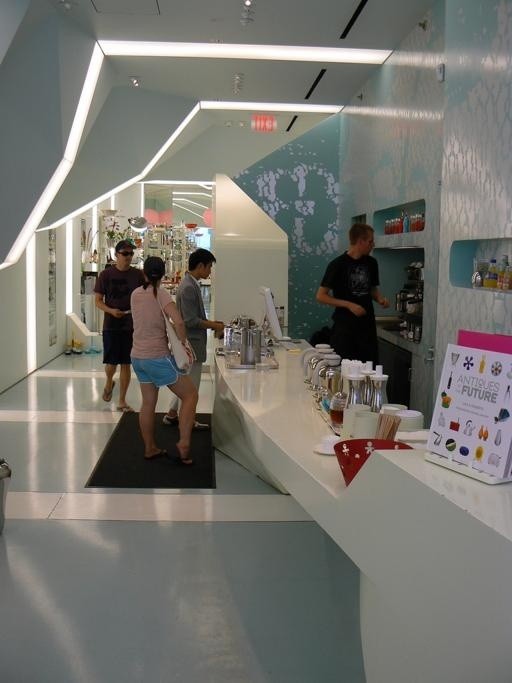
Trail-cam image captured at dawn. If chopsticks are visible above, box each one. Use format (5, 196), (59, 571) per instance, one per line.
(376, 411), (401, 441)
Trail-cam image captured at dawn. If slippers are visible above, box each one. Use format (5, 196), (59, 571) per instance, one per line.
(103, 381), (115, 401)
(143, 448), (168, 460)
(162, 454), (193, 465)
(117, 405), (134, 414)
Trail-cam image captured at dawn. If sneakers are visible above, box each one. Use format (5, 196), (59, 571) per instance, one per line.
(162, 415), (208, 429)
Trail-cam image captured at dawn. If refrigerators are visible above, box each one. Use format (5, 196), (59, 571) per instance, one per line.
(394, 264), (424, 334)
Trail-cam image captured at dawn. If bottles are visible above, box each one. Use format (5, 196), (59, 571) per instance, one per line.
(328, 358), (391, 427)
(275, 304), (285, 328)
(470, 253), (512, 291)
(384, 208), (424, 234)
(240, 329), (262, 364)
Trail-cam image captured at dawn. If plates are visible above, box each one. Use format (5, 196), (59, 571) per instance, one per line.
(315, 445), (336, 455)
(342, 403), (429, 442)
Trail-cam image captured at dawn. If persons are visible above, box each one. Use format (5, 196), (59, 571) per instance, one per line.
(162, 248), (225, 430)
(93, 240), (146, 413)
(316, 223), (391, 370)
(129, 256), (199, 466)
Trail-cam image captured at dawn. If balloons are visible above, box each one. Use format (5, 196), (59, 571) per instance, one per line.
(159, 209), (173, 225)
(203, 208), (212, 227)
(144, 209), (160, 225)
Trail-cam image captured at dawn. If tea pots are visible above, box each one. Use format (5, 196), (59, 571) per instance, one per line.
(462, 419), (476, 436)
(299, 343), (340, 397)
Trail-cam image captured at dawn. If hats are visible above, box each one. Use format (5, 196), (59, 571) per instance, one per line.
(116, 240), (136, 251)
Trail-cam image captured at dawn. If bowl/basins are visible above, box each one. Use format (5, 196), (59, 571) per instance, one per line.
(334, 438), (413, 486)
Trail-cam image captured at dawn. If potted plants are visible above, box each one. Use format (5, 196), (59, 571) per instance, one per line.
(105, 230), (124, 248)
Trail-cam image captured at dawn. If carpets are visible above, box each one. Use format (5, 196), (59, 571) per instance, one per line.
(84, 411), (216, 488)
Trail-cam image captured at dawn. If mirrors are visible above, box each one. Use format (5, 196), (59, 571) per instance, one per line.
(81, 219), (86, 323)
(48, 228), (57, 347)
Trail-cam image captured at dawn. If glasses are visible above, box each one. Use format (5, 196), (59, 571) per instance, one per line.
(117, 251), (134, 256)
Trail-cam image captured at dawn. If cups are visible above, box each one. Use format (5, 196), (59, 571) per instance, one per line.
(254, 361), (270, 374)
(322, 435), (340, 451)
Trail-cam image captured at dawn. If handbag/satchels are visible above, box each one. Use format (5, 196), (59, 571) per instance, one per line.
(163, 314), (197, 369)
